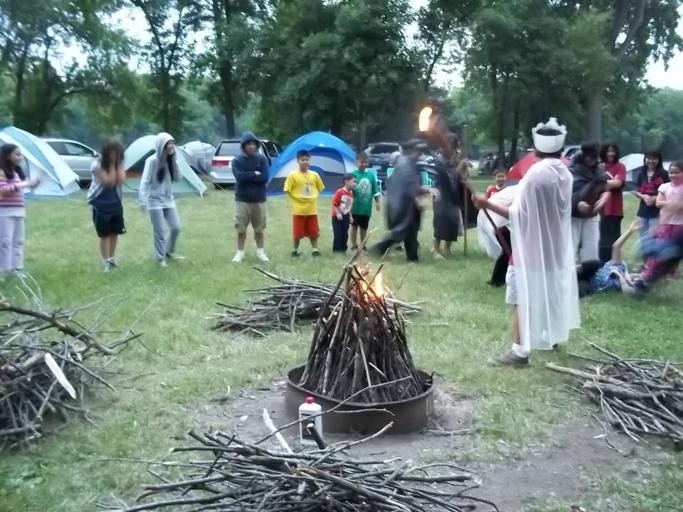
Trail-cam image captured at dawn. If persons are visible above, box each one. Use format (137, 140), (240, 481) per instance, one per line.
(505, 147), (570, 181)
(633, 160), (683, 294)
(571, 140), (603, 277)
(139, 132), (208, 267)
(284, 150), (322, 258)
(87, 139), (128, 274)
(0, 145), (41, 271)
(331, 172), (356, 252)
(231, 131), (271, 263)
(484, 170), (506, 197)
(600, 145), (626, 238)
(633, 168), (644, 186)
(348, 151), (382, 252)
(471, 129), (575, 367)
(371, 139), (431, 262)
(432, 133), (464, 260)
(631, 149), (669, 236)
(138, 132), (192, 267)
(624, 170), (636, 190)
(475, 184), (521, 286)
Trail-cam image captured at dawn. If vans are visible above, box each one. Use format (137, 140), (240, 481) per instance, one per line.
(211, 140), (283, 191)
(45, 137), (100, 187)
(364, 143), (406, 170)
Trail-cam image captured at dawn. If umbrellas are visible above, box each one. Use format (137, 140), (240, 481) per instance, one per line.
(618, 153), (645, 171)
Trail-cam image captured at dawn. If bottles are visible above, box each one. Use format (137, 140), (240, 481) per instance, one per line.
(297, 398), (322, 447)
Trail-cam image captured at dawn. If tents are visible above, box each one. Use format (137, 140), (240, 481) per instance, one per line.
(1, 125), (83, 201)
(113, 132), (206, 202)
(265, 128), (362, 200)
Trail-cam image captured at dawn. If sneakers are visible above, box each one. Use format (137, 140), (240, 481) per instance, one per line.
(232, 250), (245, 263)
(291, 251), (299, 258)
(487, 349), (529, 366)
(433, 252), (445, 261)
(157, 259), (168, 268)
(111, 257), (121, 271)
(166, 252), (184, 260)
(256, 248), (269, 262)
(312, 251), (319, 257)
(103, 259), (111, 272)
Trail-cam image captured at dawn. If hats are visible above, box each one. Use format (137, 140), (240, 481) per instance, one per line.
(531, 117), (567, 153)
